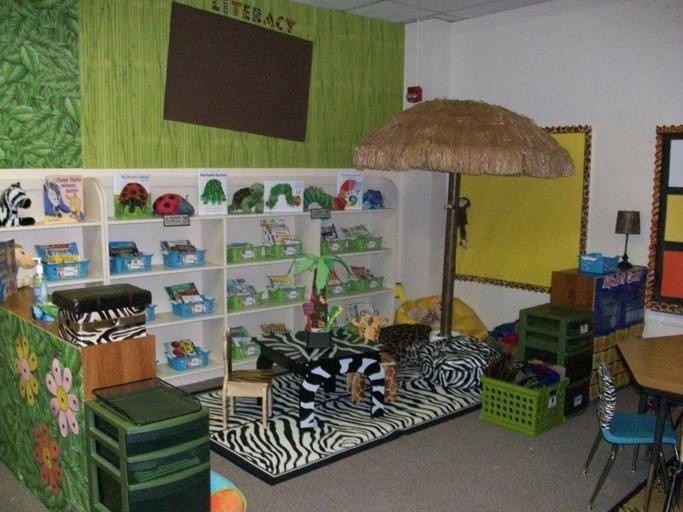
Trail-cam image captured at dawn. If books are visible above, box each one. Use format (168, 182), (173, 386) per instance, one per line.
(229, 320), (289, 363)
(343, 301), (375, 322)
(163, 282), (200, 303)
(226, 275), (298, 305)
(260, 219), (301, 256)
(319, 223), (376, 251)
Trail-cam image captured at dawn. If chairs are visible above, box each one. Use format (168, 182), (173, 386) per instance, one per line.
(220, 329), (272, 431)
(582, 359), (680, 512)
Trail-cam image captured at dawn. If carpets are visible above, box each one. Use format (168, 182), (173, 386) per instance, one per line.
(608, 454), (683, 512)
(190, 348), (482, 486)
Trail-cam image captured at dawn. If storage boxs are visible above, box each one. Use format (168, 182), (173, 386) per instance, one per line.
(345, 320), (359, 335)
(228, 346), (257, 361)
(171, 298), (215, 319)
(320, 239), (346, 256)
(51, 283), (152, 313)
(348, 275), (385, 291)
(162, 249), (207, 268)
(267, 286), (305, 304)
(347, 235), (384, 252)
(227, 245), (263, 263)
(41, 259), (90, 282)
(262, 242), (302, 259)
(167, 351), (210, 371)
(146, 304), (156, 322)
(228, 291), (264, 310)
(109, 253), (153, 272)
(325, 281), (352, 298)
(577, 253), (622, 275)
(58, 308), (147, 347)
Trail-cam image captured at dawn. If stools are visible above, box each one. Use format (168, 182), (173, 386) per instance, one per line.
(381, 324), (432, 368)
(419, 335), (500, 389)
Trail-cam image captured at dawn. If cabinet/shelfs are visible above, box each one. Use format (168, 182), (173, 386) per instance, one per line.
(84, 387), (211, 510)
(1, 170), (402, 394)
(551, 265), (647, 405)
(518, 303), (595, 419)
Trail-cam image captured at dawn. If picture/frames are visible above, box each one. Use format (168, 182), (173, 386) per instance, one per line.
(454, 125), (592, 293)
(644, 124), (683, 315)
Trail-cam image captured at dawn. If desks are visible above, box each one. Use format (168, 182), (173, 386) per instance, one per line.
(615, 334), (683, 512)
(250, 327), (387, 432)
(1, 285), (156, 509)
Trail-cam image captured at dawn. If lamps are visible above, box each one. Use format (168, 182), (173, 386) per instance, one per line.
(615, 211), (640, 269)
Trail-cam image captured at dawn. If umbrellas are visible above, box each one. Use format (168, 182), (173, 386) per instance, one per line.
(352, 98), (576, 338)
